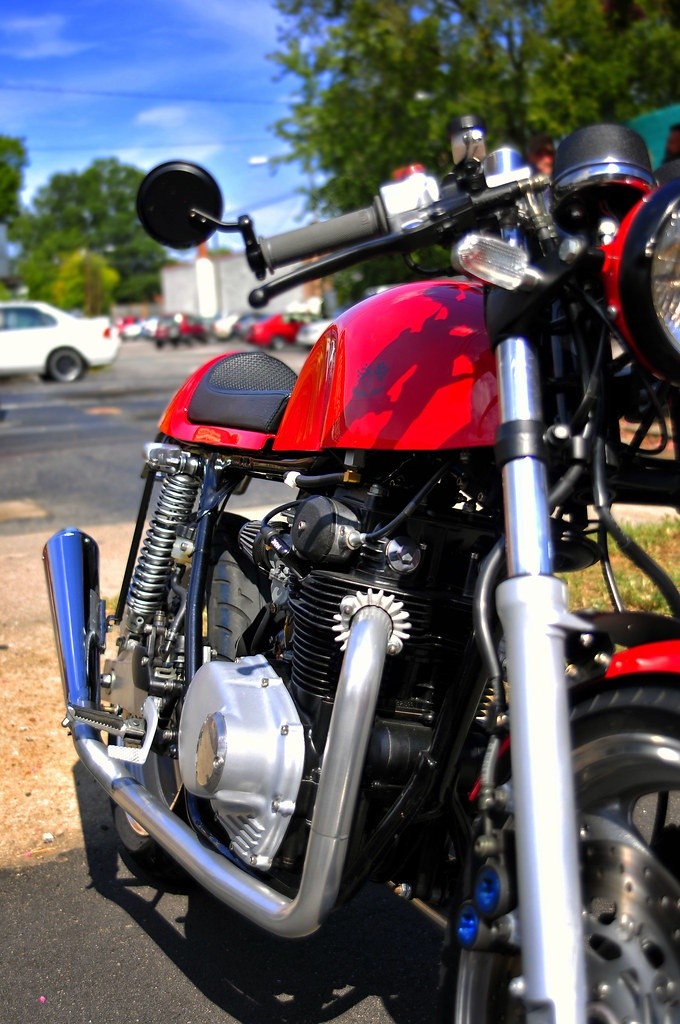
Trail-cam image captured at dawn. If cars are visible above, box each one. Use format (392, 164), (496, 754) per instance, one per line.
(114, 308), (334, 352)
(1, 300), (122, 385)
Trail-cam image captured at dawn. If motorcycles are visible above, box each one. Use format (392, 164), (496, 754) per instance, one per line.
(39, 124), (680, 1024)
